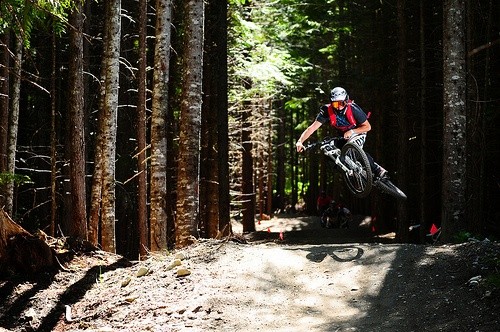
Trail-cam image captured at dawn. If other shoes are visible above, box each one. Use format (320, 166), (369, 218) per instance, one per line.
(370, 164), (378, 172)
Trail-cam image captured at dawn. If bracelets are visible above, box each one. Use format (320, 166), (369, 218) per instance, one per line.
(350, 128), (355, 134)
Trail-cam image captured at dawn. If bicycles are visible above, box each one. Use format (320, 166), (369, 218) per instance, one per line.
(300, 130), (408, 201)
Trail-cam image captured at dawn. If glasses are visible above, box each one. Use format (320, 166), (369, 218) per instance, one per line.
(331, 101), (345, 109)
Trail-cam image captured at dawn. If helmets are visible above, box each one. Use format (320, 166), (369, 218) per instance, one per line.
(330, 86), (348, 116)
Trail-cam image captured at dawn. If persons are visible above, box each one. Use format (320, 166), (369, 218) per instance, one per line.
(316, 190), (350, 230)
(295, 86), (389, 179)
